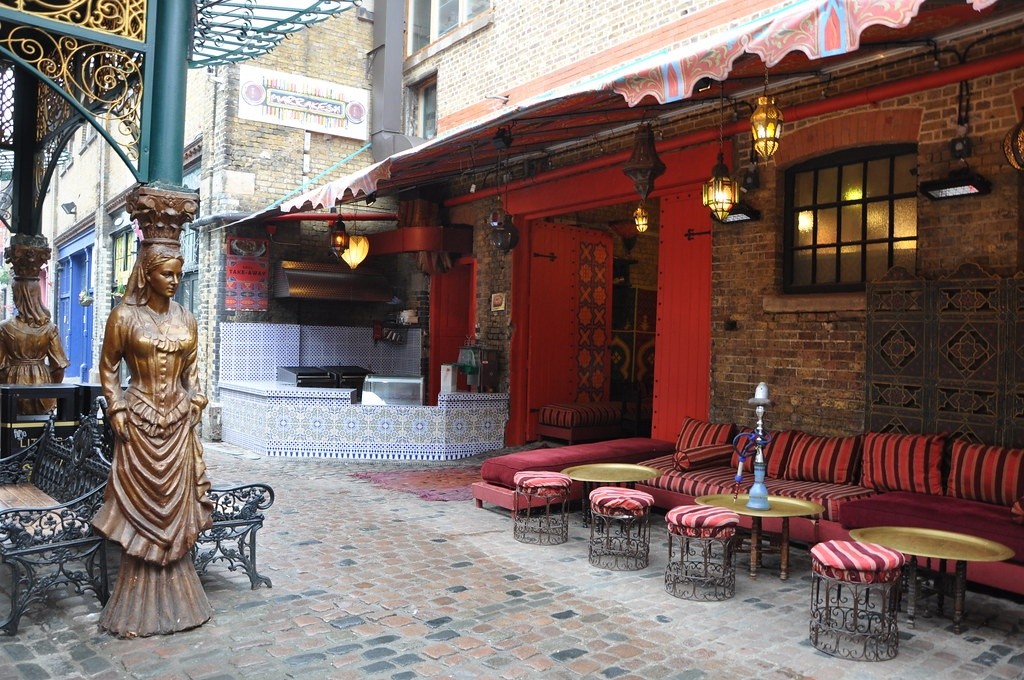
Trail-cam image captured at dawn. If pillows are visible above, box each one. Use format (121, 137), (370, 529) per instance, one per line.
(674, 416), (738, 452)
(948, 438), (1024, 505)
(673, 443), (733, 472)
(858, 428), (949, 497)
(782, 429), (871, 485)
(730, 426), (798, 479)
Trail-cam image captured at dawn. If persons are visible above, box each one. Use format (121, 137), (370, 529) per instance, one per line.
(0, 234), (71, 415)
(88, 188), (215, 639)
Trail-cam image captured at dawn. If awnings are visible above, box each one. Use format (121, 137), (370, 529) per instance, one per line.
(208, 0), (1024, 232)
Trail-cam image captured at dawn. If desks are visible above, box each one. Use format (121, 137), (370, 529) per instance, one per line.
(560, 461), (660, 534)
(849, 526), (1015, 633)
(697, 494), (825, 578)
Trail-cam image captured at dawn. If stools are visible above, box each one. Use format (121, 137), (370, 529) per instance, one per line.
(809, 539), (906, 661)
(664, 505), (739, 602)
(512, 470), (571, 545)
(589, 487), (654, 570)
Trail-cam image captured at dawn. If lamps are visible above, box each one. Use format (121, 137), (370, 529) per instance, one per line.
(711, 171), (759, 223)
(919, 136), (992, 201)
(702, 82), (739, 221)
(633, 198), (648, 232)
(327, 202), (350, 258)
(751, 64), (784, 166)
(341, 203), (369, 270)
(61, 202), (76, 214)
(366, 194), (376, 206)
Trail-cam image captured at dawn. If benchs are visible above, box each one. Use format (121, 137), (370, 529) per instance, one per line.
(0, 409), (117, 637)
(536, 402), (636, 446)
(620, 452), (1024, 597)
(472, 438), (676, 520)
(91, 395), (273, 605)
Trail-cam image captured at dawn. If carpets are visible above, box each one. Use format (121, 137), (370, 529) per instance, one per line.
(347, 464), (481, 502)
